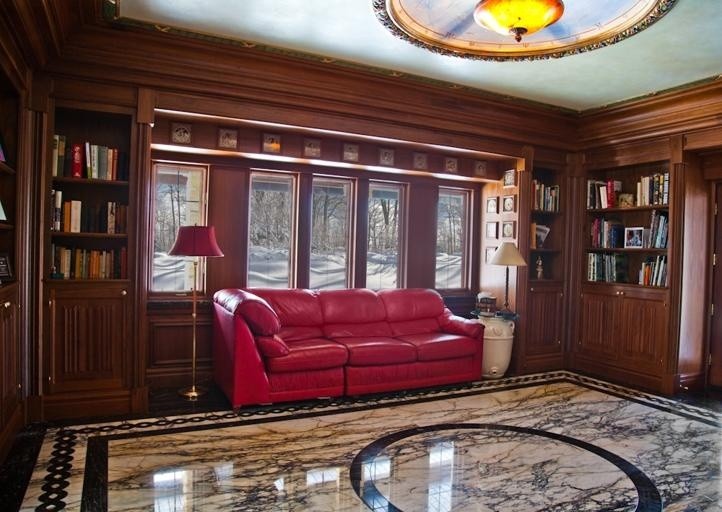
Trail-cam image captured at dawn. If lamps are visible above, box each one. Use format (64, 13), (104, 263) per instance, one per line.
(168, 220), (226, 401)
(471, 0), (565, 43)
(487, 241), (528, 313)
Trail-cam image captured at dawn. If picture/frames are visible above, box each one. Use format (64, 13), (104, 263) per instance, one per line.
(503, 168), (517, 188)
(485, 195), (516, 264)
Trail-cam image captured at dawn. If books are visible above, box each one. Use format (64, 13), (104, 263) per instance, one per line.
(530, 176), (562, 214)
(578, 169), (672, 286)
(43, 126), (131, 282)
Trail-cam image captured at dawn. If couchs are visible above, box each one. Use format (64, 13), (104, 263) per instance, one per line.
(209, 286), (485, 416)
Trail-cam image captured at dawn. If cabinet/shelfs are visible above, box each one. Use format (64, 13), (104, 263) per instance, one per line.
(572, 144), (712, 397)
(33, 87), (155, 426)
(517, 159), (568, 373)
